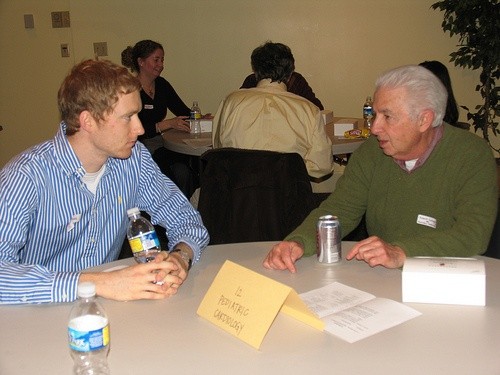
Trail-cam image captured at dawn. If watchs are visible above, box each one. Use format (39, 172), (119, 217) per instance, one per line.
(168, 249), (192, 271)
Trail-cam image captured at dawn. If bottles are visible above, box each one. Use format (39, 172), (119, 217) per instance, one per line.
(362, 96), (374, 131)
(67, 281), (112, 375)
(124, 207), (170, 292)
(190, 101), (201, 137)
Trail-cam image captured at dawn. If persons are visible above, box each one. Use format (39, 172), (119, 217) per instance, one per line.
(239, 72), (324, 111)
(121, 40), (214, 202)
(210, 40), (334, 178)
(0, 57), (211, 304)
(264, 64), (498, 274)
(421, 60), (458, 125)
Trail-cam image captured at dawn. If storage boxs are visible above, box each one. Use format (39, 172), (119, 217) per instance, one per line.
(334, 118), (358, 136)
(322, 110), (334, 125)
(190, 119), (213, 133)
(401, 255), (487, 307)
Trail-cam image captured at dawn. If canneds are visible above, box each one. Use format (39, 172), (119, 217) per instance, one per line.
(316, 214), (342, 263)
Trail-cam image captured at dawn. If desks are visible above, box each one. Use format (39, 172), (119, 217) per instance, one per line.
(0, 238), (500, 375)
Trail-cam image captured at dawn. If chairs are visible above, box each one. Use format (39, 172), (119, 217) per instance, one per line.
(196, 145), (318, 240)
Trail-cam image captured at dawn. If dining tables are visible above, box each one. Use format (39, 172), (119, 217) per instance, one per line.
(160, 115), (375, 156)
